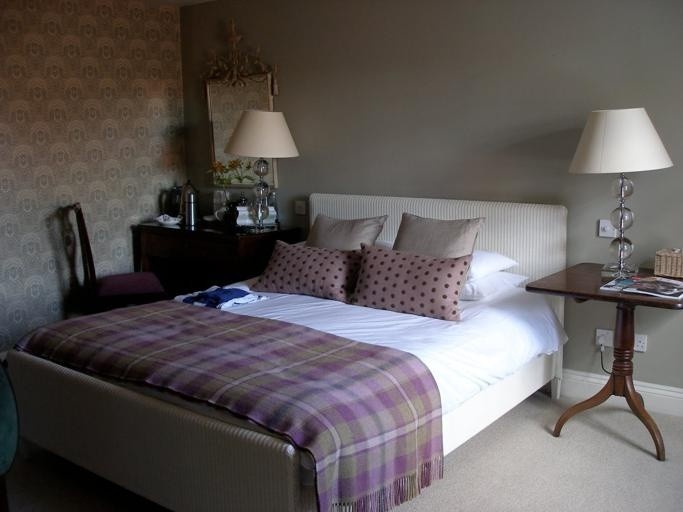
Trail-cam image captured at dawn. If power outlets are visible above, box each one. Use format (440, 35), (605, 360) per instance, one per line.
(593, 327), (613, 348)
(633, 334), (648, 355)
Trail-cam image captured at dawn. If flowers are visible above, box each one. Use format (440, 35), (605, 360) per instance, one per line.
(223, 156), (255, 185)
(208, 161), (233, 203)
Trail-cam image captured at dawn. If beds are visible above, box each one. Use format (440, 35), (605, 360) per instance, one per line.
(3, 187), (572, 510)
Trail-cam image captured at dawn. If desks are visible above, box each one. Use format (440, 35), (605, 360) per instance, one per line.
(130, 215), (303, 295)
(524, 262), (682, 462)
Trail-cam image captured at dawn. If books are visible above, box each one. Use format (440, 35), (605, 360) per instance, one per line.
(600, 275), (683, 300)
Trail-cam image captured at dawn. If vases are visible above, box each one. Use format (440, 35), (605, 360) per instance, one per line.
(223, 198), (239, 225)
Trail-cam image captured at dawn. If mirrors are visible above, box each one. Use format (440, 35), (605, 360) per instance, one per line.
(196, 16), (283, 192)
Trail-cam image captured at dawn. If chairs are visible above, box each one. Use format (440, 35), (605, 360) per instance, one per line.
(68, 201), (166, 314)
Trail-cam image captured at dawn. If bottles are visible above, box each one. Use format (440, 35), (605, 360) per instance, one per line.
(209, 176), (280, 232)
(158, 178), (202, 226)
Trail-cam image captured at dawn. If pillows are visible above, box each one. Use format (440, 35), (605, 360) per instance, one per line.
(302, 209), (390, 257)
(454, 268), (528, 302)
(392, 208), (484, 265)
(250, 235), (358, 307)
(461, 246), (521, 282)
(346, 240), (475, 324)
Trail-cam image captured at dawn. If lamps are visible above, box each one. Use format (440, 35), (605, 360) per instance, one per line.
(221, 105), (301, 234)
(565, 105), (674, 281)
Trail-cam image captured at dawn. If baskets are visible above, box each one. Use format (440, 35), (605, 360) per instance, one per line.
(652, 248), (682, 278)
